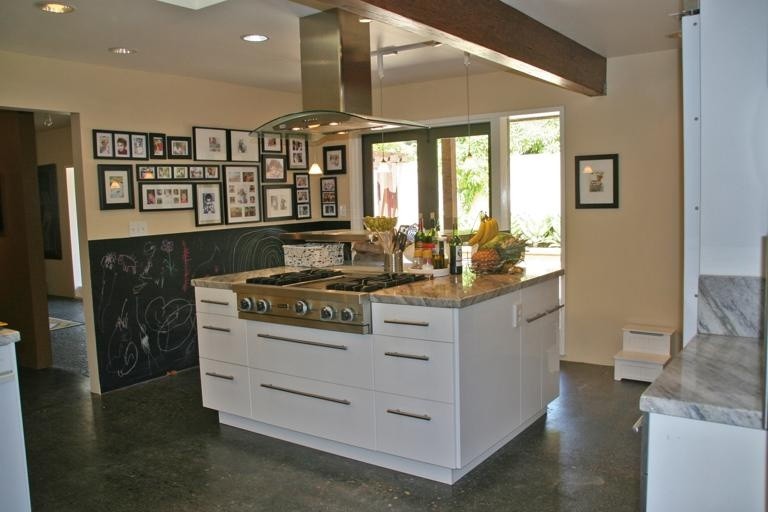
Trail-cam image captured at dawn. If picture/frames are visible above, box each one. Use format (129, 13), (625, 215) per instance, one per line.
(573, 152), (620, 210)
(91, 125), (348, 228)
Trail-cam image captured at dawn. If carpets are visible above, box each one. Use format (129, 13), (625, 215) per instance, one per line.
(48, 317), (84, 331)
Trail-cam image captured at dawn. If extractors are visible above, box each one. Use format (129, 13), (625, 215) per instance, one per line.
(248, 7), (430, 134)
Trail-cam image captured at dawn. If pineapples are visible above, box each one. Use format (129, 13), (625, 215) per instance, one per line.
(472, 233), (530, 270)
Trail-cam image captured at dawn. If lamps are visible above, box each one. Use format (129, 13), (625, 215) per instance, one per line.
(376, 54), (391, 175)
(464, 54), (474, 167)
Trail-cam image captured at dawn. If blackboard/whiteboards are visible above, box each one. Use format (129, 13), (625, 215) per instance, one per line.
(87, 220), (352, 395)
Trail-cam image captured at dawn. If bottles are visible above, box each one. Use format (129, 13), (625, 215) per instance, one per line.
(413, 217), (462, 275)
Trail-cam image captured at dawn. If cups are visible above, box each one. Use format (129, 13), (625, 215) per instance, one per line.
(384, 252), (403, 272)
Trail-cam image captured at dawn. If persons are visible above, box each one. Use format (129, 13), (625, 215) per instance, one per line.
(100, 136), (339, 217)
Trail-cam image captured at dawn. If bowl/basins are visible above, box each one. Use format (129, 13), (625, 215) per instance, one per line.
(468, 258), (524, 275)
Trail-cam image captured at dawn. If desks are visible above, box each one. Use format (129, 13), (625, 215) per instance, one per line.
(194, 276), (564, 485)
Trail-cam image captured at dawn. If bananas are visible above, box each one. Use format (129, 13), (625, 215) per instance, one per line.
(468, 211), (498, 246)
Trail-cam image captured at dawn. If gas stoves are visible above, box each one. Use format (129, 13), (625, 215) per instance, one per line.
(231, 268), (433, 335)
